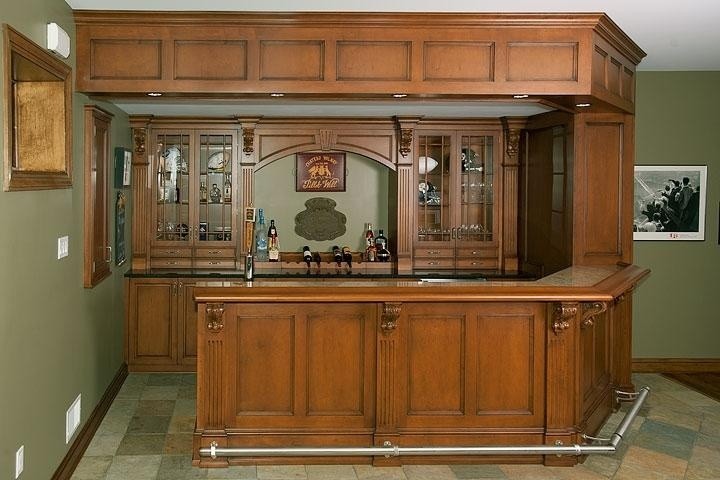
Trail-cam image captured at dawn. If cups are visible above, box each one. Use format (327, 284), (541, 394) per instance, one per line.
(418, 223), (488, 242)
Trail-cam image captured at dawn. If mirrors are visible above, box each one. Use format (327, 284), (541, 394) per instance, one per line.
(2, 22), (72, 194)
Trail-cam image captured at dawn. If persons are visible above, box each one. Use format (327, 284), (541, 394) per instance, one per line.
(634, 176), (701, 232)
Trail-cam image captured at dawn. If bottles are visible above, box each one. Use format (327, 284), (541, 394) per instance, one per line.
(255, 207), (390, 268)
(223, 175), (233, 205)
(198, 180), (207, 202)
(244, 247), (254, 282)
(171, 180), (180, 202)
(210, 182), (222, 203)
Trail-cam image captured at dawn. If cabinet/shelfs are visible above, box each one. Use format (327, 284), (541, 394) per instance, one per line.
(411, 120), (504, 276)
(124, 114), (249, 372)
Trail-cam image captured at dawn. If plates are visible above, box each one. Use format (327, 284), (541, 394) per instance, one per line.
(206, 150), (231, 171)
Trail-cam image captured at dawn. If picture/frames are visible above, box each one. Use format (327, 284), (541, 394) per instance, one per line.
(634, 163), (709, 242)
